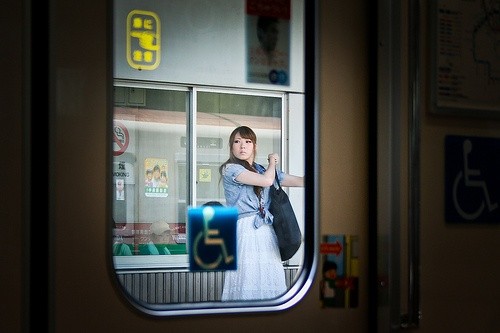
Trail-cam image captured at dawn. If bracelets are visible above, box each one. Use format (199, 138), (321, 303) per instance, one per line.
(269, 156), (277, 163)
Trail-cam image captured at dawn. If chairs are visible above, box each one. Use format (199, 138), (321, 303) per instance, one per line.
(114, 242), (186, 255)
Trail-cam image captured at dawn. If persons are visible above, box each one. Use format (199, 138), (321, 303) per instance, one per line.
(218, 126), (305, 301)
(146, 165), (168, 189)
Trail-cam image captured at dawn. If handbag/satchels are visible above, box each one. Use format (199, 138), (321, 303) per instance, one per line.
(268, 185), (301, 261)
(148, 219), (171, 236)
